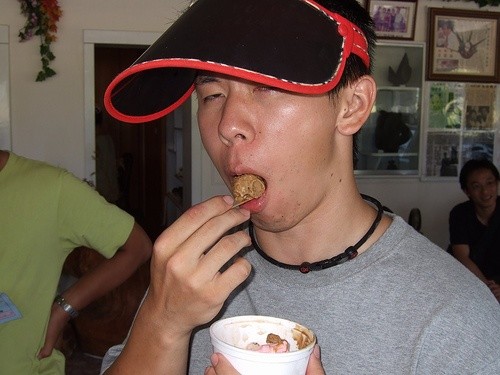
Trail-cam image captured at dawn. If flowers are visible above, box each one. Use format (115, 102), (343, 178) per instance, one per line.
(17, 0), (63, 82)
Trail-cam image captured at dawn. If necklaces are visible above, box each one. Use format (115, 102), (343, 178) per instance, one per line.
(249, 193), (384, 272)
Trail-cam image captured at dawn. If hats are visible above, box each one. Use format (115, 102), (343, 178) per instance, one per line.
(103, 0), (370, 123)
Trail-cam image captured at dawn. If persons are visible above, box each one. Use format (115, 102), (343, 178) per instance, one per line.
(0, 150), (152, 375)
(99, 0), (500, 375)
(445, 158), (500, 304)
(373, 7), (405, 32)
(436, 20), (460, 51)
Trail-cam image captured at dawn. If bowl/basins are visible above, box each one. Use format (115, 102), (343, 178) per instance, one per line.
(210, 315), (316, 375)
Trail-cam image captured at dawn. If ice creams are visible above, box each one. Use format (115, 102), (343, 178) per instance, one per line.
(245, 332), (289, 354)
(231, 172), (267, 202)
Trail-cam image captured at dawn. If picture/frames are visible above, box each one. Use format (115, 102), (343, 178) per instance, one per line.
(353, 0), (500, 84)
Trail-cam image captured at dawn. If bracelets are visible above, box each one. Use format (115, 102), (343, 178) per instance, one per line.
(55, 296), (78, 318)
